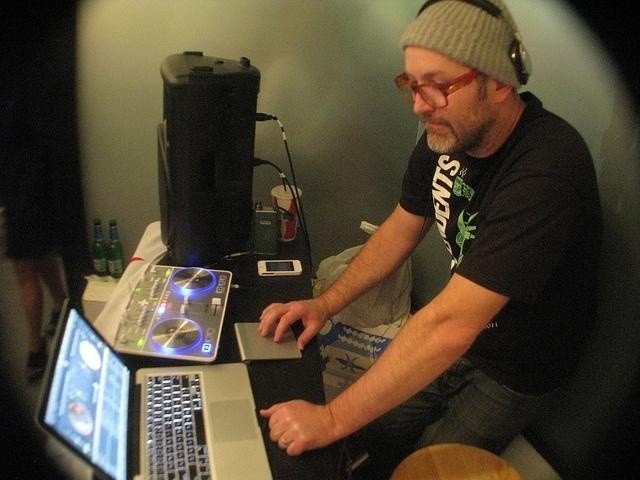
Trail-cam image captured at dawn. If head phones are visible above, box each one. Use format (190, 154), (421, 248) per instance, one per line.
(414, 0), (532, 84)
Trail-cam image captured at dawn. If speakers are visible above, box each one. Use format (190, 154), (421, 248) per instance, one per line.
(158, 52), (260, 266)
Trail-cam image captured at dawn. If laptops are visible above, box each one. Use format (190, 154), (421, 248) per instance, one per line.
(34, 297), (273, 480)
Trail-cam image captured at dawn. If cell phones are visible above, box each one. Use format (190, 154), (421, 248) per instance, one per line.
(257, 259), (303, 277)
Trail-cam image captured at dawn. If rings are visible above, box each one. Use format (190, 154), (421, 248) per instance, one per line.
(280, 436), (290, 445)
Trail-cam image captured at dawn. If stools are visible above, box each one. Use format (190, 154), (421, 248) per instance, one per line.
(387, 443), (521, 479)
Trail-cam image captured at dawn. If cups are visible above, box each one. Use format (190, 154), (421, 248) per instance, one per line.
(270, 184), (303, 243)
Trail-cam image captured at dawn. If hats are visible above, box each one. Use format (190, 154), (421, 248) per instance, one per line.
(400, 0), (523, 88)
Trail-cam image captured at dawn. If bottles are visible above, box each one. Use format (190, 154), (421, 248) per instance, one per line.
(91, 218), (125, 279)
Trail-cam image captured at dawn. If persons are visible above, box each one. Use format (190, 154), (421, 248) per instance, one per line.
(259, 0), (601, 466)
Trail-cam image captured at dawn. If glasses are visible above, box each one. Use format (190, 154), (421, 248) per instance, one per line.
(395, 68), (481, 110)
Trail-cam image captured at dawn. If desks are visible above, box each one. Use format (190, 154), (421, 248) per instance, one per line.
(45, 219), (336, 480)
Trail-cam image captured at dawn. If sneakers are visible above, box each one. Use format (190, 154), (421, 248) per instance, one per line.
(26, 338), (48, 382)
(44, 305), (61, 336)
